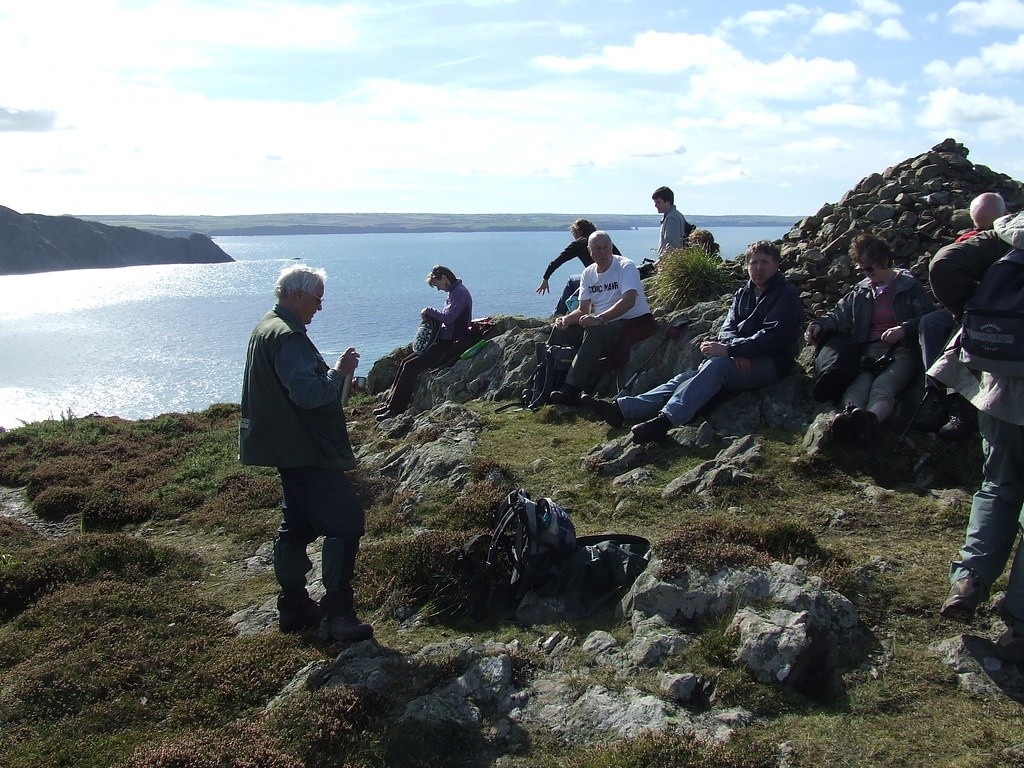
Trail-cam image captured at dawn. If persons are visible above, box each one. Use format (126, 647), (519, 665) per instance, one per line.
(536, 219), (622, 319)
(529, 230), (657, 411)
(918, 192), (1024, 624)
(237, 270), (374, 641)
(802, 233), (936, 446)
(579, 241), (804, 441)
(373, 266), (472, 423)
(652, 186), (722, 262)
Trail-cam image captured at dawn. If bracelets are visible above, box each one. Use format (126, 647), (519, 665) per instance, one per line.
(597, 314), (607, 323)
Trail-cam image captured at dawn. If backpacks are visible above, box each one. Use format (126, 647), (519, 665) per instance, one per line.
(480, 489), (575, 604)
(959, 250), (1024, 360)
(527, 342), (576, 412)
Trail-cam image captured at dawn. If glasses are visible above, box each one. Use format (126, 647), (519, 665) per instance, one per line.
(854, 266), (873, 273)
(301, 288), (324, 303)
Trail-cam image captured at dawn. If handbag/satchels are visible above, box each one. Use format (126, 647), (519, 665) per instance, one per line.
(412, 321), (442, 356)
(637, 257), (655, 280)
(684, 221), (696, 239)
(809, 329), (863, 403)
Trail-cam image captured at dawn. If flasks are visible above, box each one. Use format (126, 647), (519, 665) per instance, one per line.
(460, 340), (486, 361)
(337, 351), (356, 406)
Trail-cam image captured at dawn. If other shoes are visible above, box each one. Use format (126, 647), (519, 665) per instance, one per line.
(850, 407), (880, 437)
(939, 576), (980, 614)
(376, 407), (407, 421)
(549, 386), (577, 404)
(630, 412), (674, 442)
(938, 415), (963, 436)
(373, 406), (388, 415)
(831, 405), (852, 446)
(278, 597), (321, 628)
(318, 610), (374, 641)
(591, 397), (625, 429)
(991, 621), (1024, 659)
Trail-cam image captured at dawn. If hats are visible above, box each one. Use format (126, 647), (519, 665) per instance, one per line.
(992, 208), (1024, 251)
(969, 192), (1006, 228)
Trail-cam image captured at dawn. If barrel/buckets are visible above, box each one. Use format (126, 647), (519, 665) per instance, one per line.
(351, 377), (366, 393)
